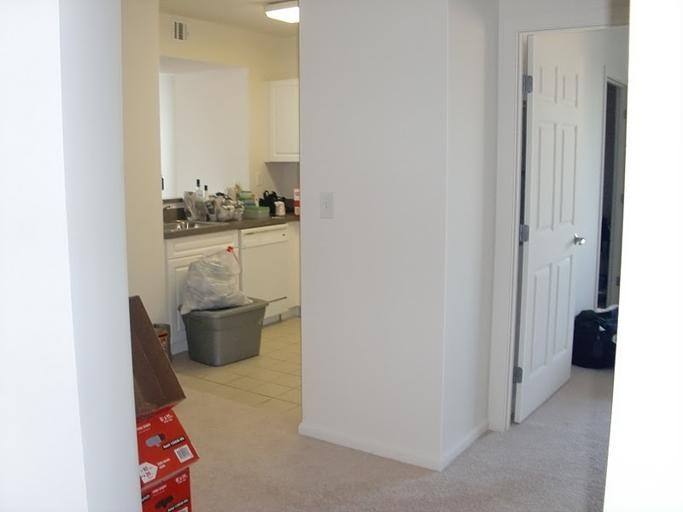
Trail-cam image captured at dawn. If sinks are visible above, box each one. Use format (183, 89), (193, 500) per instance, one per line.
(163, 220), (229, 234)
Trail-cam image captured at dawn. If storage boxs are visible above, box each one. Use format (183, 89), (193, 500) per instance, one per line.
(177, 295), (269, 368)
(130, 295), (201, 511)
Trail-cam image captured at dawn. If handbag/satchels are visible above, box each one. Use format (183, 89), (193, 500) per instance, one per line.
(571, 308), (617, 369)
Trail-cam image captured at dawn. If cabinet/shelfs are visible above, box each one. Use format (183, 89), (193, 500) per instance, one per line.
(164, 224), (240, 358)
(264, 79), (299, 162)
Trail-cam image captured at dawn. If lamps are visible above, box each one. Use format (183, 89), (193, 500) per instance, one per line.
(264, 0), (300, 26)
